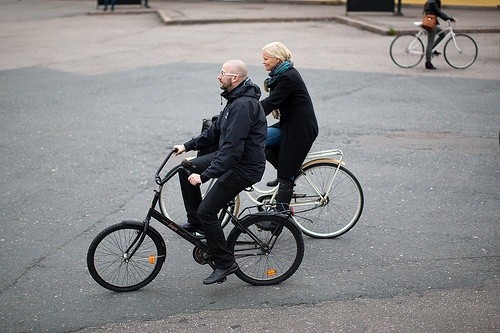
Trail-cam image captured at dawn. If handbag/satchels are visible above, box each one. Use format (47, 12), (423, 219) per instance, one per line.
(423, 14), (437, 27)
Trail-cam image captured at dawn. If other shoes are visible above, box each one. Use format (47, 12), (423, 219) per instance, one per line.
(255, 222), (280, 232)
(266, 180), (283, 186)
(423, 61), (436, 69)
(431, 50), (442, 56)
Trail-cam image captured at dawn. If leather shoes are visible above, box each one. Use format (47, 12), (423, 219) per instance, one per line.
(203, 260), (238, 286)
(178, 221), (197, 231)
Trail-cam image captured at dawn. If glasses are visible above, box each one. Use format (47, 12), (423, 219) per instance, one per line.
(220, 70), (239, 78)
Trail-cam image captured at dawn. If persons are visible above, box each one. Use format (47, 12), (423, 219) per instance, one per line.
(423, 0), (458, 70)
(255, 42), (318, 235)
(172, 59), (267, 284)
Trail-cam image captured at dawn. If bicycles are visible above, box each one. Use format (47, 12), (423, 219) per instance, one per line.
(158, 147), (365, 240)
(389, 21), (479, 69)
(86, 148), (305, 293)
